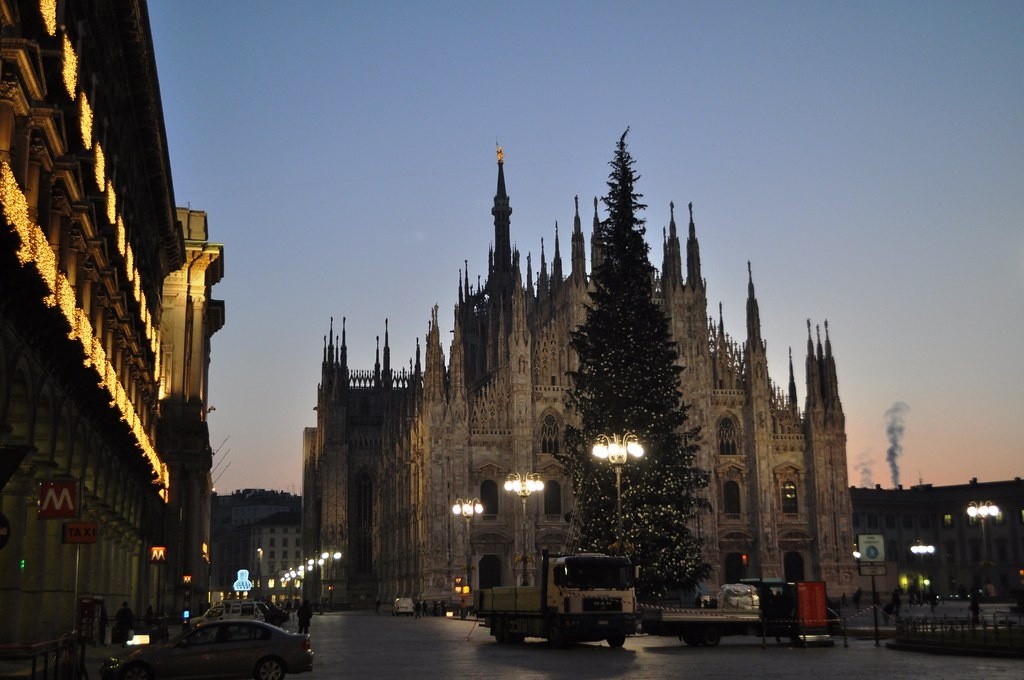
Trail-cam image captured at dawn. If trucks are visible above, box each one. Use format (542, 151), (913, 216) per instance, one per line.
(642, 581), (839, 648)
(472, 549), (637, 651)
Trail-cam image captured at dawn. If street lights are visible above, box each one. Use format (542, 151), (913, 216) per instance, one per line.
(504, 472), (545, 587)
(452, 497), (484, 593)
(298, 564), (314, 601)
(967, 500), (1000, 561)
(280, 570), (304, 600)
(910, 545), (935, 580)
(321, 551), (343, 610)
(592, 432), (645, 557)
(307, 558), (325, 603)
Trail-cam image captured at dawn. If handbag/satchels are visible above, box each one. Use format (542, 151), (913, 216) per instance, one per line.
(127, 626), (134, 640)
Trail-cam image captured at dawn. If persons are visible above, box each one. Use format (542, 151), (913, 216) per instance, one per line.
(100, 601), (108, 645)
(199, 599), (212, 615)
(694, 592), (718, 607)
(854, 584), (990, 618)
(297, 601), (313, 634)
(413, 599), (467, 619)
(114, 601), (133, 649)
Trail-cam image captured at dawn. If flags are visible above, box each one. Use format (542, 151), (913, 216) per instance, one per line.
(496, 146), (503, 162)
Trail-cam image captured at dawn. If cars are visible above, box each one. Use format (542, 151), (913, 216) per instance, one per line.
(99, 619), (314, 680)
(392, 598), (416, 616)
(189, 600), (290, 634)
